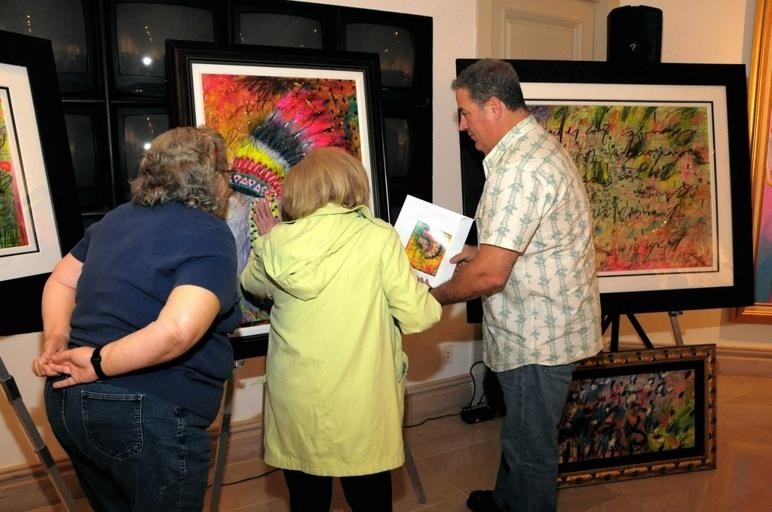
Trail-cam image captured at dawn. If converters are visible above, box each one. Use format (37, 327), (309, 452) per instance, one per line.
(460, 404), (495, 424)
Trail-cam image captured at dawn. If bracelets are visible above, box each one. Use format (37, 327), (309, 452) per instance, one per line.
(91, 344), (106, 378)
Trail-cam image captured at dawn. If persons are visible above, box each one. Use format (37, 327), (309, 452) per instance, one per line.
(30, 123), (243, 511)
(240, 145), (442, 512)
(429, 56), (605, 511)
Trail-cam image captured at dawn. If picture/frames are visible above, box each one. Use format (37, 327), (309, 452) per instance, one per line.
(0, 29), (89, 346)
(454, 54), (758, 328)
(163, 34), (399, 365)
(552, 340), (720, 492)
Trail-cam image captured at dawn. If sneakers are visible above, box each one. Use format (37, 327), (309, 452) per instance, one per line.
(466, 489), (496, 512)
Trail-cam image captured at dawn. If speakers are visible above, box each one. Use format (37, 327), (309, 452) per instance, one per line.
(606, 4), (664, 62)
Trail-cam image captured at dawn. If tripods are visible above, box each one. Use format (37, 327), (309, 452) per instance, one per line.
(601, 311), (658, 352)
